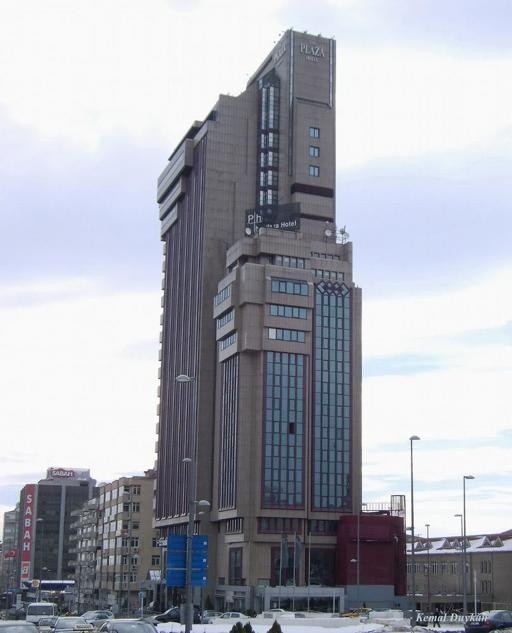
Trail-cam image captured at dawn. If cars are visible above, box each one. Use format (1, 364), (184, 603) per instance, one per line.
(464, 609), (512, 633)
(0, 603), (289, 633)
(339, 607), (462, 620)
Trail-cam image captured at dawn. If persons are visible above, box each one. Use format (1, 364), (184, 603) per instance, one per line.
(432, 607), (440, 627)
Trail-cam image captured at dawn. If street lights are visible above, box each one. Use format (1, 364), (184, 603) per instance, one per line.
(173, 370), (211, 632)
(38, 566), (47, 603)
(407, 432), (476, 616)
